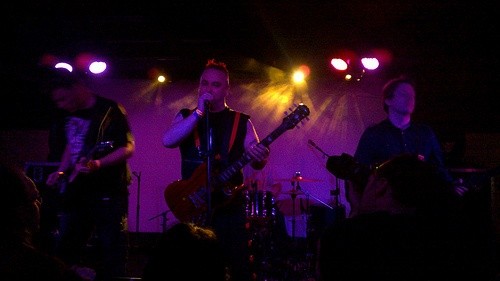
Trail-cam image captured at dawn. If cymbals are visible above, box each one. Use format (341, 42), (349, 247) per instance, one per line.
(274, 177), (325, 183)
(279, 189), (306, 195)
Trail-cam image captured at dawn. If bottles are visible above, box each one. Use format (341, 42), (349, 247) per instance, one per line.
(271, 196), (276, 216)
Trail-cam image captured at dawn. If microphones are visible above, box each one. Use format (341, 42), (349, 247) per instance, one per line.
(203, 99), (210, 107)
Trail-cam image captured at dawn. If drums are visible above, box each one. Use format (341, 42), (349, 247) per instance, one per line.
(242, 190), (276, 220)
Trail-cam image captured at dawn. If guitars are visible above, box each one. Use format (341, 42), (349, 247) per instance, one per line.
(24, 141), (114, 208)
(164, 104), (310, 229)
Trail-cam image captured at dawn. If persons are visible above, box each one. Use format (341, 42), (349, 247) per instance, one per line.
(344, 76), (443, 217)
(143, 221), (225, 281)
(0, 166), (83, 281)
(162, 62), (270, 281)
(47, 78), (138, 281)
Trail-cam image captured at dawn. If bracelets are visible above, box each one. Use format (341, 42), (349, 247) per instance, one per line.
(95, 160), (100, 168)
(192, 109), (203, 119)
